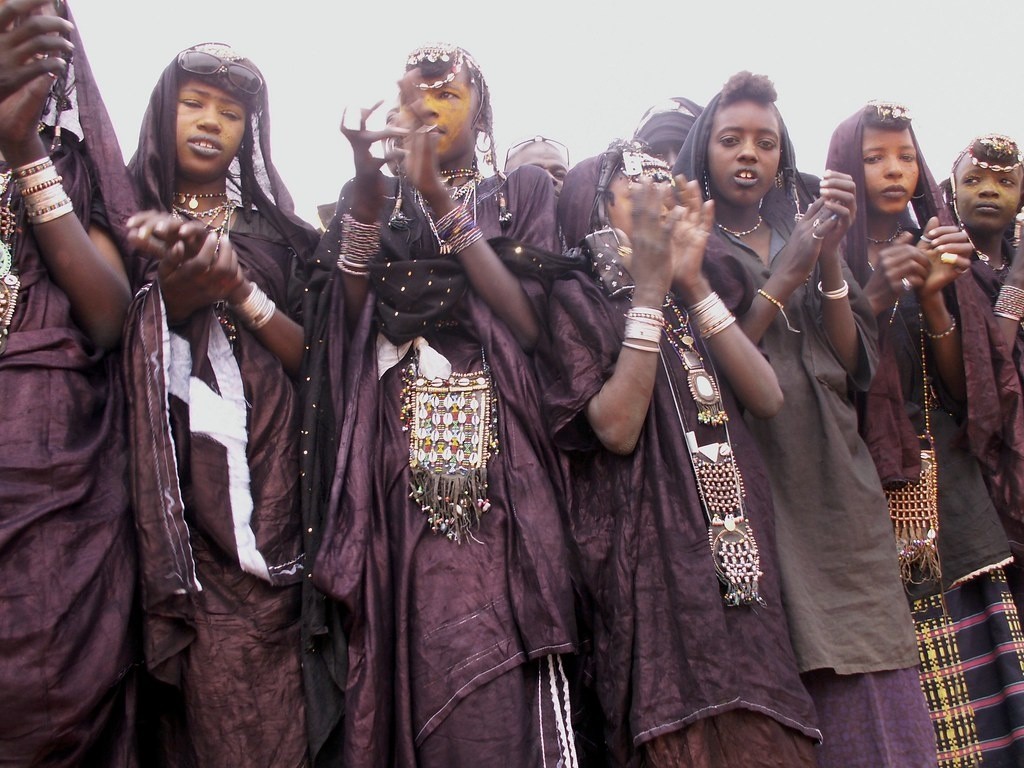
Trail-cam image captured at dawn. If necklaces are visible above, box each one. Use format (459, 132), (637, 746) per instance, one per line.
(411, 166), (483, 246)
(867, 220), (903, 244)
(0, 121), (45, 281)
(661, 288), (730, 427)
(716, 214), (763, 239)
(171, 190), (237, 341)
(984, 255), (1007, 271)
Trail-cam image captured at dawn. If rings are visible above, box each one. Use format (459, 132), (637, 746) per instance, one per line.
(954, 267), (969, 274)
(812, 233), (824, 240)
(901, 277), (913, 291)
(617, 245), (632, 257)
(813, 219), (820, 228)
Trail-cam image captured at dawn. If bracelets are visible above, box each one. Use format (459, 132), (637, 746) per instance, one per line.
(336, 212), (382, 275)
(992, 285), (1023, 321)
(925, 313), (956, 339)
(13, 156), (75, 224)
(756, 289), (800, 333)
(226, 280), (276, 330)
(818, 279), (848, 299)
(436, 205), (485, 255)
(687, 291), (736, 340)
(620, 306), (665, 353)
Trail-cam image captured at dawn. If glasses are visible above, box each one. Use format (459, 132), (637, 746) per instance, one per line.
(636, 98), (698, 118)
(503, 135), (569, 170)
(179, 50), (262, 96)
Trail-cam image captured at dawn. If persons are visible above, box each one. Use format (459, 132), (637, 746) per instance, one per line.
(304, 38), (590, 768)
(824, 98), (1023, 767)
(669, 71), (944, 768)
(937, 131), (1024, 391)
(530, 136), (831, 768)
(503, 131), (572, 206)
(0, 0), (138, 768)
(123, 40), (322, 768)
(629, 96), (706, 171)
